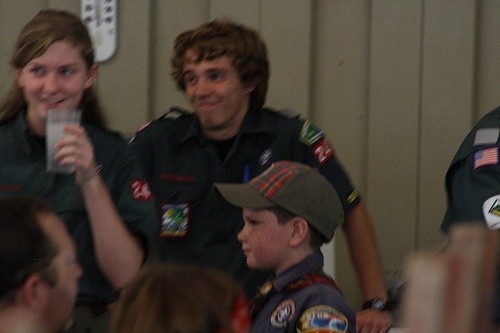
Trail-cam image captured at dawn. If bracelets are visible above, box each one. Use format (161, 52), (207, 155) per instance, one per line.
(74, 161), (105, 185)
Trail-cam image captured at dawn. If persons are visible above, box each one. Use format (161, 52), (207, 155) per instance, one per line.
(1, 0), (393, 333)
(439, 105), (500, 333)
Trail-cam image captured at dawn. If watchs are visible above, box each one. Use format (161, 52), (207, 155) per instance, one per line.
(360, 296), (393, 311)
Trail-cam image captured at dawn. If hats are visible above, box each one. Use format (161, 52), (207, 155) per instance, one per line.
(214, 162), (345, 241)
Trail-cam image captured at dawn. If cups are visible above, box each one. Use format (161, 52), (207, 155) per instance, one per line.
(45, 108), (82, 175)
